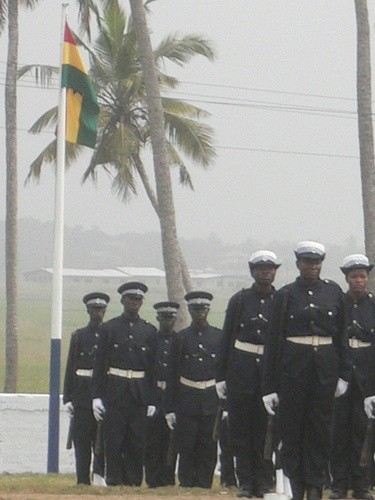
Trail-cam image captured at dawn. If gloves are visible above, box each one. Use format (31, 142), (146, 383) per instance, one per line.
(147, 406), (155, 416)
(64, 402), (74, 417)
(334, 378), (347, 396)
(164, 413), (175, 430)
(222, 411), (227, 420)
(91, 398), (105, 421)
(216, 381), (227, 400)
(363, 396), (375, 419)
(262, 393), (278, 415)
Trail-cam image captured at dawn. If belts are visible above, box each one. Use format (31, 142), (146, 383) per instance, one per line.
(349, 338), (370, 348)
(286, 335), (332, 346)
(76, 368), (92, 376)
(108, 367), (145, 379)
(234, 340), (265, 355)
(180, 376), (215, 389)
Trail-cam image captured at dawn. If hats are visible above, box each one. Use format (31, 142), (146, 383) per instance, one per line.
(293, 241), (325, 260)
(82, 292), (110, 308)
(153, 302), (179, 317)
(184, 291), (212, 308)
(248, 250), (281, 268)
(339, 254), (373, 274)
(117, 281), (148, 300)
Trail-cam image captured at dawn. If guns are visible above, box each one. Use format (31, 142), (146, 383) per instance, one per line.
(94, 420), (103, 456)
(264, 415), (273, 461)
(66, 418), (75, 451)
(167, 428), (177, 459)
(359, 421), (374, 467)
(212, 401), (226, 443)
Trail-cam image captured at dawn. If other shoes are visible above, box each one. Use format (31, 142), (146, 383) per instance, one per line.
(329, 489), (347, 500)
(352, 489), (374, 500)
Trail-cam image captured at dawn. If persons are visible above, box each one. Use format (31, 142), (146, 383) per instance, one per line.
(329, 254), (375, 500)
(215, 250), (282, 498)
(262, 241), (353, 500)
(89, 282), (157, 487)
(161, 291), (240, 489)
(63, 291), (110, 486)
(145, 301), (180, 489)
